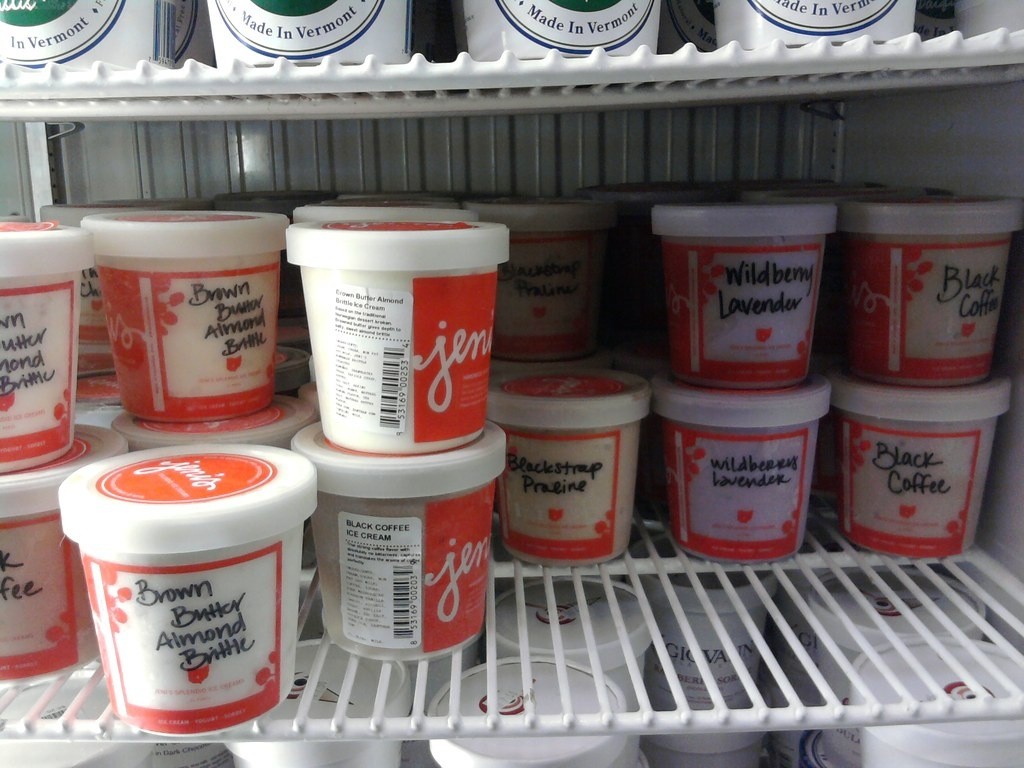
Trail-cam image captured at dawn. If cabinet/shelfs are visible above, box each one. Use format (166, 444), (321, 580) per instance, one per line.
(0, 0), (1024, 768)
